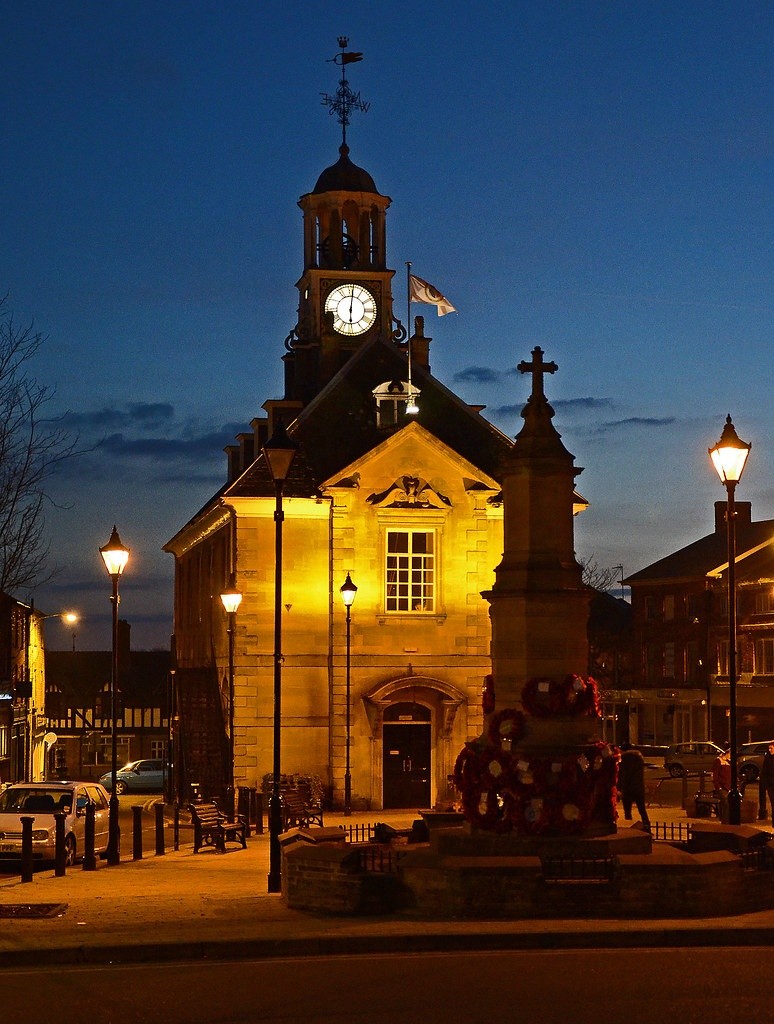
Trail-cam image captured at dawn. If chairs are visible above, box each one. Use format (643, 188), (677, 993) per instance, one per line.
(24, 793), (87, 811)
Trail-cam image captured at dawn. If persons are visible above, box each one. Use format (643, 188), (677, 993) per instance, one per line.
(713, 740), (730, 823)
(755, 742), (774, 827)
(616, 741), (654, 835)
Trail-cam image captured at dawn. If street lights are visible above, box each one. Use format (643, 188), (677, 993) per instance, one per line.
(340, 572), (359, 817)
(707, 411), (751, 827)
(98, 522), (130, 865)
(218, 573), (244, 842)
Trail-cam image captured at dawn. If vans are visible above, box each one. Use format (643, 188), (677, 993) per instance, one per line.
(664, 739), (724, 778)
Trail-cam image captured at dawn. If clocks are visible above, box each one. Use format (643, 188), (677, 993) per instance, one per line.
(324, 284), (377, 336)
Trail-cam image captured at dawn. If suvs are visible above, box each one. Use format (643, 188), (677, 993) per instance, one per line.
(735, 741), (774, 783)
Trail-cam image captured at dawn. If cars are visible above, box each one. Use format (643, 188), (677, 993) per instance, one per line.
(0, 779), (122, 866)
(99, 759), (183, 796)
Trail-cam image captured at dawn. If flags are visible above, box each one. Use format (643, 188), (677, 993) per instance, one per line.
(409, 275), (459, 318)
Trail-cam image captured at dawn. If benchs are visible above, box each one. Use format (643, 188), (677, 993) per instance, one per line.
(694, 781), (748, 821)
(189, 801), (247, 854)
(282, 794), (323, 833)
